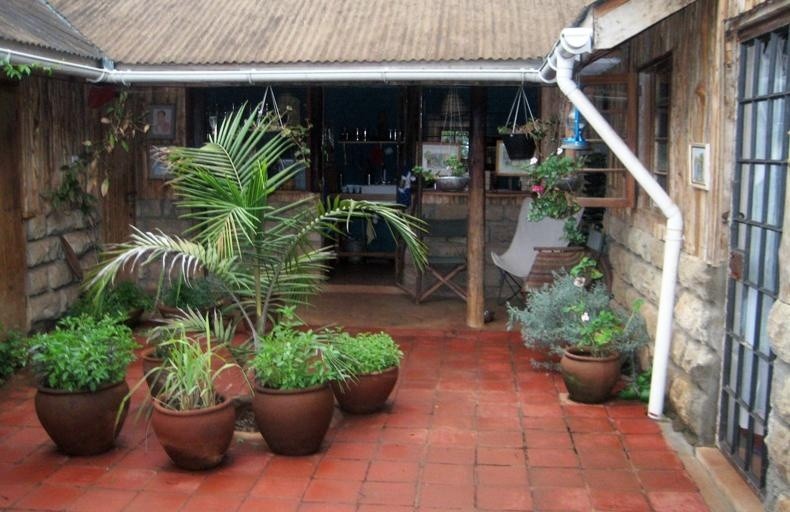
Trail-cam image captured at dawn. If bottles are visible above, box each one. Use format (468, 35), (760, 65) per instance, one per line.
(338, 124), (403, 142)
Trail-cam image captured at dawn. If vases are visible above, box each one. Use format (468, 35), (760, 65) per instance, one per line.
(560, 346), (621, 404)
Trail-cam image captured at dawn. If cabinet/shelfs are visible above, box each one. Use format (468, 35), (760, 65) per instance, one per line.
(331, 136), (403, 274)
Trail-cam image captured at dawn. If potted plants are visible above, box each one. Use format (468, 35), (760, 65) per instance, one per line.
(497, 118), (552, 160)
(141, 325), (185, 400)
(113, 322), (254, 470)
(248, 304), (352, 456)
(233, 104), (313, 170)
(323, 329), (405, 415)
(63, 276), (285, 334)
(412, 156), (472, 192)
(8, 308), (145, 457)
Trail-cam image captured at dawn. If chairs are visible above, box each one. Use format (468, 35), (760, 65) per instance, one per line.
(415, 216), (468, 305)
(490, 195), (585, 304)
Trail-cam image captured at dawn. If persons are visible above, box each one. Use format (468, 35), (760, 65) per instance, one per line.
(152, 111), (170, 133)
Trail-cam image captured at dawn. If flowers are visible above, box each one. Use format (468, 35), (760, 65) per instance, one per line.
(560, 255), (645, 357)
(525, 153), (589, 242)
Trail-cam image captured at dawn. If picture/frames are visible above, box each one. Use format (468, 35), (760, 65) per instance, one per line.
(687, 143), (711, 191)
(145, 103), (176, 140)
(146, 142), (178, 180)
(495, 140), (538, 176)
(415, 141), (460, 176)
